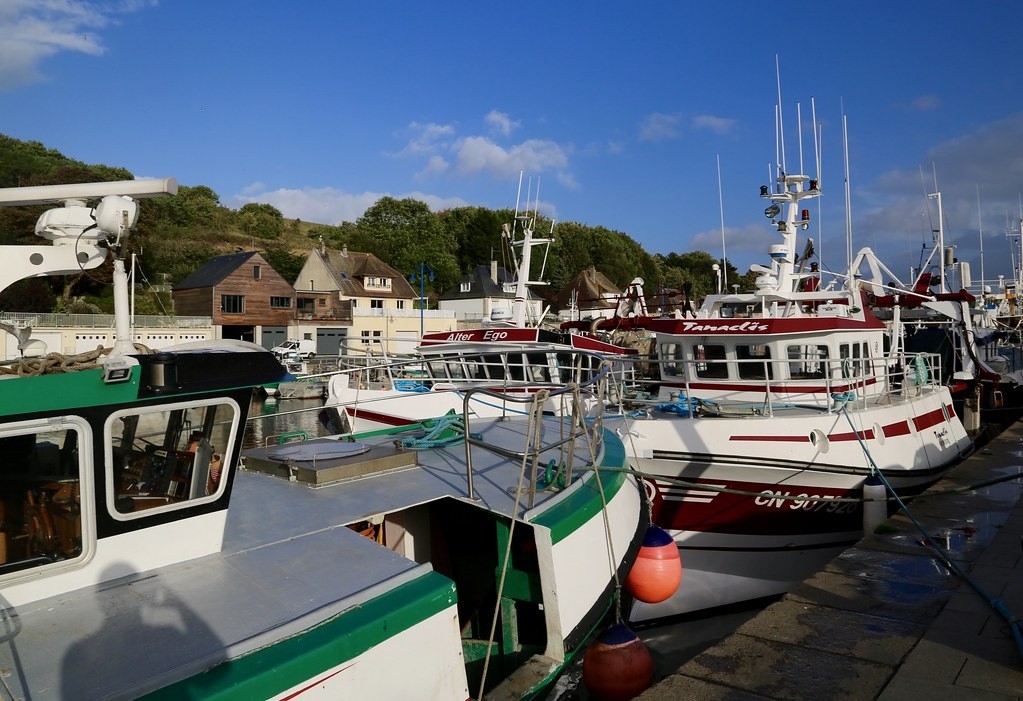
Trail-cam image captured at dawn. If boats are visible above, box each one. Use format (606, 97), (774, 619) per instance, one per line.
(1, 180), (654, 701)
(564, 51), (1000, 628)
(318, 172), (638, 440)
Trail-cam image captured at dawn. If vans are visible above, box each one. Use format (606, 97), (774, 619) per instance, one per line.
(272, 339), (317, 358)
